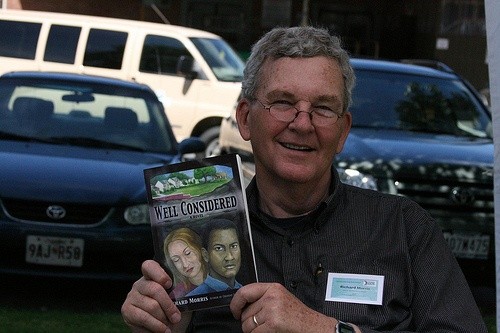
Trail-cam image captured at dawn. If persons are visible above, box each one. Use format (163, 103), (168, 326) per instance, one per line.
(119, 26), (488, 333)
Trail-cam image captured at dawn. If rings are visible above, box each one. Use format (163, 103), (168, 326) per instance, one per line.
(251, 315), (260, 327)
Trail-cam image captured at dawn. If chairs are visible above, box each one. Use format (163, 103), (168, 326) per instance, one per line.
(13, 96), (55, 137)
(101, 107), (139, 143)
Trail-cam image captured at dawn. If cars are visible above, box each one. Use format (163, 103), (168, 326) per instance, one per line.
(0, 71), (208, 311)
(332, 58), (495, 260)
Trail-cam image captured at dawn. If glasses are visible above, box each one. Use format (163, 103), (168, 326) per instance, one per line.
(254, 97), (343, 128)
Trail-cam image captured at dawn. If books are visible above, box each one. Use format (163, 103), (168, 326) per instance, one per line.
(143, 153), (259, 314)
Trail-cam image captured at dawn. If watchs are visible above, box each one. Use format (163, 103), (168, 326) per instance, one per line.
(335, 319), (356, 333)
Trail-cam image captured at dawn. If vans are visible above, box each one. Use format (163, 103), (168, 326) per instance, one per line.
(0, 9), (254, 162)
